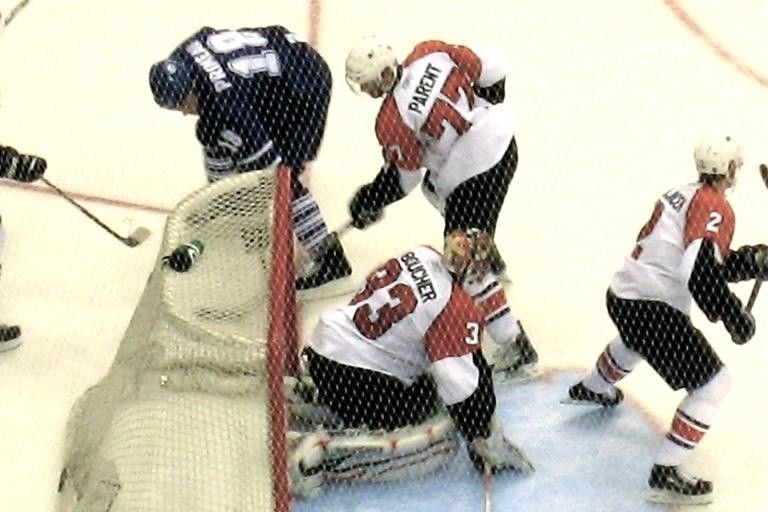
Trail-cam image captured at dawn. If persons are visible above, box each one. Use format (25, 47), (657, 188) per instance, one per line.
(149, 25), (350, 288)
(306, 226), (528, 472)
(560, 135), (767, 505)
(346, 36), (538, 372)
(0, 144), (48, 351)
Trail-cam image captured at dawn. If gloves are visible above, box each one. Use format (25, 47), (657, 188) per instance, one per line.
(739, 243), (768, 282)
(471, 430), (535, 475)
(1, 145), (48, 184)
(721, 294), (758, 344)
(349, 183), (386, 229)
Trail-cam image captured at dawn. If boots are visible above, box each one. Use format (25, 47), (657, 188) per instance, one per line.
(638, 460), (714, 508)
(291, 235), (360, 303)
(0, 322), (22, 353)
(485, 329), (541, 383)
(557, 378), (627, 409)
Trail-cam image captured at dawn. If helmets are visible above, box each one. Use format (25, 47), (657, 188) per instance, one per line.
(343, 43), (399, 100)
(149, 59), (194, 110)
(440, 228), (507, 285)
(692, 133), (746, 177)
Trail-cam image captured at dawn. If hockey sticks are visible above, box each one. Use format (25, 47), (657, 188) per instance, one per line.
(31, 170), (152, 250)
(746, 161), (768, 324)
